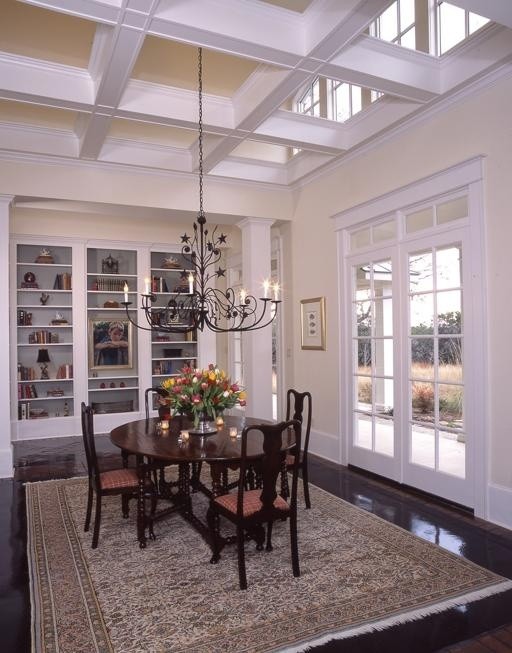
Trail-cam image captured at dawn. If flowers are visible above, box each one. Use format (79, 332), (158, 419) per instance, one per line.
(157, 362), (250, 429)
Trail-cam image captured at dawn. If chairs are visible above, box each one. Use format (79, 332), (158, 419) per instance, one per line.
(143, 385), (213, 503)
(252, 389), (312, 509)
(81, 400), (155, 549)
(208, 419), (304, 588)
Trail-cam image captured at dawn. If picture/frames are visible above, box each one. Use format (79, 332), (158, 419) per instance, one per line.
(300, 297), (327, 351)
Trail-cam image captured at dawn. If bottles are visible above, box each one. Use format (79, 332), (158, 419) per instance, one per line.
(64, 403), (70, 416)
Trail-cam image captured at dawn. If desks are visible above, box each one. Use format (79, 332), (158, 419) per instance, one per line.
(106, 414), (297, 547)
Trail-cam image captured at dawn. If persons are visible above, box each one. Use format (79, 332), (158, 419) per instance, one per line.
(95, 321), (129, 365)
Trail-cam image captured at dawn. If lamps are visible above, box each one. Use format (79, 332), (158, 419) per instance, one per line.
(120, 38), (283, 333)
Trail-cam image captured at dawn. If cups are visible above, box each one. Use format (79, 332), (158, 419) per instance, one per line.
(223, 386), (246, 431)
(63, 362), (70, 379)
(54, 311), (64, 321)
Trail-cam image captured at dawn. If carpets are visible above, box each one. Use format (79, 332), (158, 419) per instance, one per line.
(25, 467), (512, 653)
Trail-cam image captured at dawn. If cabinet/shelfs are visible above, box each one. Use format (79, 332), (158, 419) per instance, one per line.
(9, 234), (199, 440)
